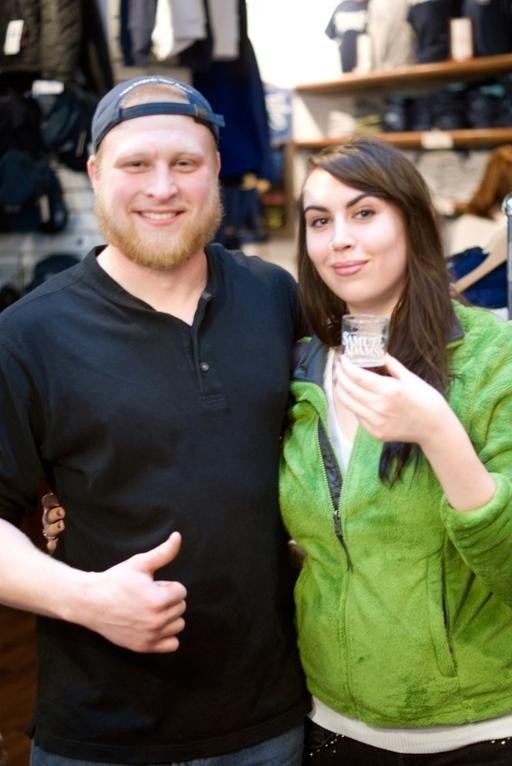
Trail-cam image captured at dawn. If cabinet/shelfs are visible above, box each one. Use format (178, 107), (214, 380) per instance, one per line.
(258, 55), (508, 239)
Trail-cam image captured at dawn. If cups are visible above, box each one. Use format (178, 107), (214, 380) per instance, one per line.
(341, 315), (390, 369)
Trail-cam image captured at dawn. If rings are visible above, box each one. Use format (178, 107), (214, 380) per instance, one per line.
(42, 526), (56, 540)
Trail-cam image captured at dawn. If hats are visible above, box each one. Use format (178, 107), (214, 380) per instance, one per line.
(91, 74), (225, 155)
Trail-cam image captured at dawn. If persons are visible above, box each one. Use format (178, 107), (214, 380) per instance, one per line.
(40, 136), (512, 766)
(0, 74), (314, 766)
